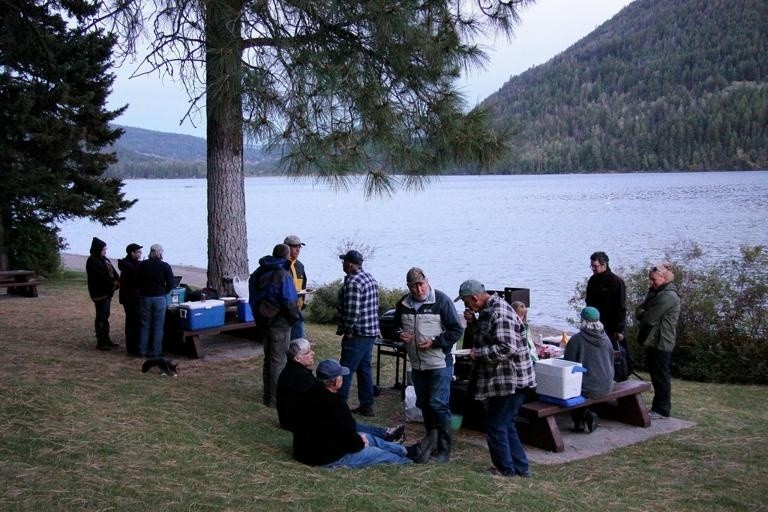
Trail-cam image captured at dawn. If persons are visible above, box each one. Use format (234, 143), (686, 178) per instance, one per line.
(583, 250), (634, 379)
(510, 298), (537, 365)
(248, 243), (301, 409)
(563, 305), (616, 433)
(334, 250), (380, 418)
(454, 279), (538, 476)
(85, 237), (121, 354)
(282, 234), (308, 342)
(635, 261), (680, 419)
(276, 337), (407, 444)
(391, 268), (464, 466)
(118, 243), (143, 358)
(290, 356), (440, 469)
(136, 243), (174, 358)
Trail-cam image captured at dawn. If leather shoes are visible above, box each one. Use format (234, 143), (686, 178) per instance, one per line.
(350, 404), (377, 419)
(395, 433), (407, 446)
(585, 411), (599, 433)
(411, 428), (438, 464)
(431, 454), (449, 464)
(384, 424), (405, 442)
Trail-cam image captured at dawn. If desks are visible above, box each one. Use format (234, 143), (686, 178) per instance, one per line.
(0, 266), (39, 282)
(373, 340), (408, 401)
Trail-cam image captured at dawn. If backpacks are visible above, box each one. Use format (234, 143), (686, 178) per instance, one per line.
(613, 338), (644, 384)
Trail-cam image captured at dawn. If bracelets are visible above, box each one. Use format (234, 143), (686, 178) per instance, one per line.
(431, 336), (437, 345)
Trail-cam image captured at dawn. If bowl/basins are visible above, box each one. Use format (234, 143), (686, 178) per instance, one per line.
(167, 275), (184, 288)
(449, 414), (465, 432)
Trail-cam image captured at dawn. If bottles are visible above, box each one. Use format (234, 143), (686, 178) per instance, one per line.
(200, 292), (206, 303)
(560, 331), (569, 346)
(534, 332), (544, 354)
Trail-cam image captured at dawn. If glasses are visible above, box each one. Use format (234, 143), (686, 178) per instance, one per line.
(589, 263), (602, 270)
(649, 267), (662, 275)
(135, 250), (142, 254)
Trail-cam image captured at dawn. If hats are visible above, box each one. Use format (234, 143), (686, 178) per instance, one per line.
(284, 235), (306, 248)
(406, 267), (426, 287)
(338, 250), (364, 265)
(453, 279), (486, 302)
(580, 306), (601, 323)
(316, 359), (351, 380)
(126, 243), (144, 254)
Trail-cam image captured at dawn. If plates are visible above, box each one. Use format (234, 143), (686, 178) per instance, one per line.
(448, 348), (475, 358)
(296, 288), (316, 296)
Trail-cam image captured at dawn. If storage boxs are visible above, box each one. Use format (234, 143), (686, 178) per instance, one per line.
(534, 356), (589, 409)
(175, 299), (229, 333)
(164, 286), (187, 311)
(235, 296), (258, 324)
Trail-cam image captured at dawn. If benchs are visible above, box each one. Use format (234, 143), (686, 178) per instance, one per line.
(0, 279), (42, 300)
(514, 376), (655, 454)
(165, 319), (260, 360)
